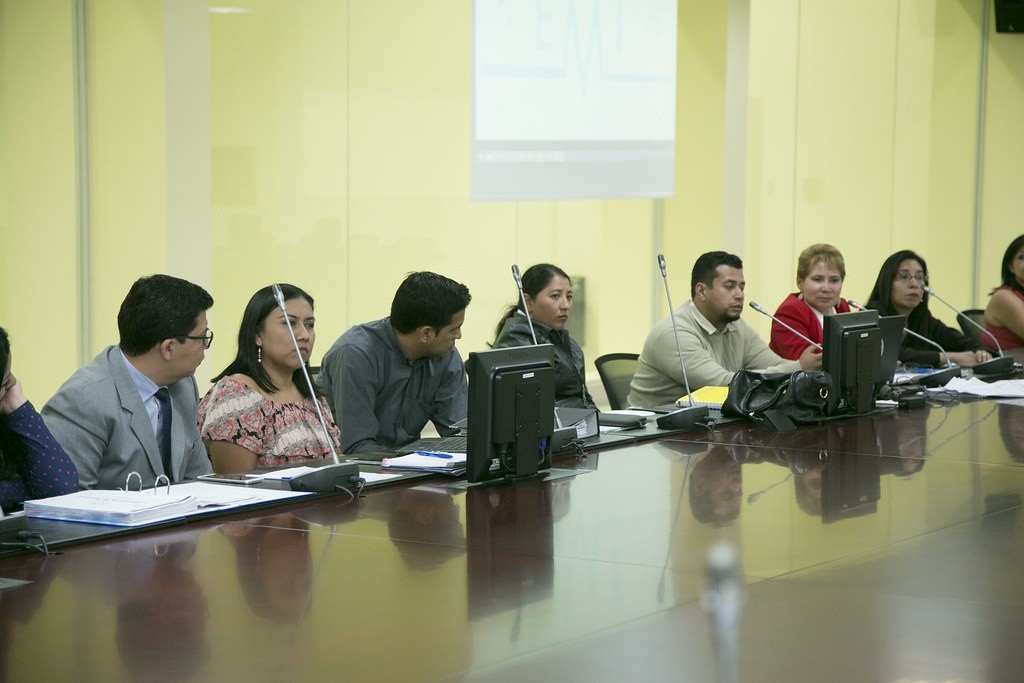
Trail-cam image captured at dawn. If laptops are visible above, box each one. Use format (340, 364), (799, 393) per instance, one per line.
(878, 314), (906, 386)
(394, 436), (467, 456)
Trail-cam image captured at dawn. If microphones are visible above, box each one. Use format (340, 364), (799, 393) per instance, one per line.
(512, 265), (578, 452)
(920, 284), (1014, 375)
(847, 300), (962, 388)
(272, 284), (360, 492)
(654, 254), (710, 429)
(749, 302), (823, 351)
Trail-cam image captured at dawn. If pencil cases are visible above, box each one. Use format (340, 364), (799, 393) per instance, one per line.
(600, 413), (647, 428)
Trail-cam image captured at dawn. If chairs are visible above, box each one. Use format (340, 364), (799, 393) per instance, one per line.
(957, 309), (985, 343)
(595, 354), (639, 409)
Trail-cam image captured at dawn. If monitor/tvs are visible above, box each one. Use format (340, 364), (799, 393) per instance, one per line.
(821, 310), (881, 415)
(465, 342), (555, 485)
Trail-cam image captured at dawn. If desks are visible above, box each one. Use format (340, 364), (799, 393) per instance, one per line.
(0, 376), (1024, 683)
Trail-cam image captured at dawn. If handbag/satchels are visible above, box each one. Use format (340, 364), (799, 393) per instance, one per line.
(721, 369), (834, 424)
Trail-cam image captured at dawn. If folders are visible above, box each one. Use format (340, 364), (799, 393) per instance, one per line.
(449, 408), (600, 442)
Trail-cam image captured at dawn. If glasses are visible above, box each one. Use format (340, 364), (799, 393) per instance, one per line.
(896, 271), (928, 284)
(161, 328), (213, 349)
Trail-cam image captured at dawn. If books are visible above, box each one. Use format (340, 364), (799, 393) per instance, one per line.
(676, 386), (728, 408)
(23, 472), (316, 526)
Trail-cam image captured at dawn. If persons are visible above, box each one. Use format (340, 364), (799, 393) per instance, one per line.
(980, 234), (1024, 349)
(487, 264), (600, 412)
(860, 250), (1000, 368)
(626, 251), (822, 409)
(0, 328), (79, 516)
(40, 274), (215, 491)
(316, 273), (472, 455)
(769, 245), (853, 361)
(196, 283), (341, 474)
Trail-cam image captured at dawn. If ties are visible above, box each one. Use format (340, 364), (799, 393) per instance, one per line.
(154, 388), (174, 484)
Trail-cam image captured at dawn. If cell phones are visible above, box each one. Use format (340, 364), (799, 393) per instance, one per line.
(196, 473), (264, 485)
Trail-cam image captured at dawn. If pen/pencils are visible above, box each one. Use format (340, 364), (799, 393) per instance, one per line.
(417, 450), (453, 459)
